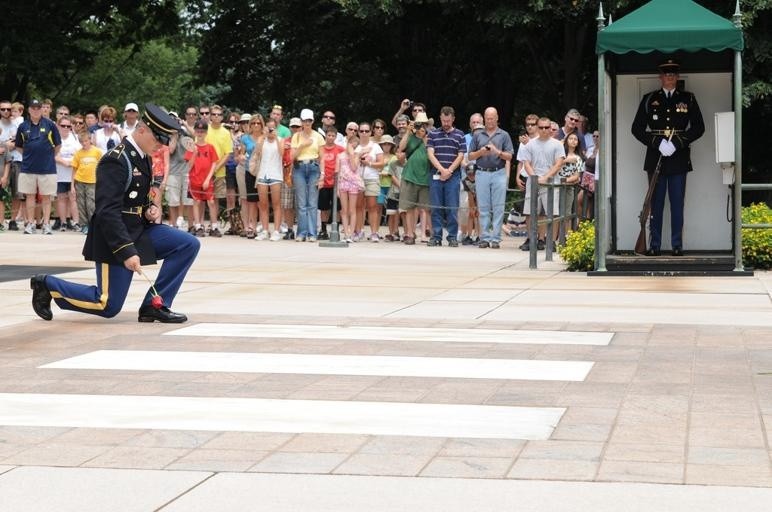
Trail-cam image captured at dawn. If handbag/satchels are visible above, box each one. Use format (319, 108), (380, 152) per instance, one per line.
(229, 207), (243, 234)
(379, 172), (393, 188)
(580, 171), (596, 193)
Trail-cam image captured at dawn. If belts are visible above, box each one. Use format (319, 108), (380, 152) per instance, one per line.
(475, 166), (507, 173)
(650, 128), (686, 136)
(122, 205), (147, 215)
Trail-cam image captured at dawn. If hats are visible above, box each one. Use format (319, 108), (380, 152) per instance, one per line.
(143, 104), (181, 147)
(238, 112), (252, 122)
(378, 134), (397, 148)
(126, 101), (140, 113)
(466, 162), (474, 175)
(289, 107), (315, 128)
(410, 113), (434, 127)
(657, 58), (683, 75)
(27, 98), (42, 107)
(193, 119), (207, 129)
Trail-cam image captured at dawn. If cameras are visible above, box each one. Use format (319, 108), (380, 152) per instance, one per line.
(407, 101), (414, 106)
(178, 120), (184, 125)
(105, 123), (113, 128)
(268, 129), (274, 133)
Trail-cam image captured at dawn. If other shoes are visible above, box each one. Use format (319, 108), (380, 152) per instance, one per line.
(185, 230), (501, 252)
(519, 238), (529, 250)
(537, 236), (547, 249)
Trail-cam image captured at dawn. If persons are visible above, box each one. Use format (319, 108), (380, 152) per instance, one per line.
(30, 102), (201, 324)
(0, 98), (600, 254)
(631, 55), (705, 256)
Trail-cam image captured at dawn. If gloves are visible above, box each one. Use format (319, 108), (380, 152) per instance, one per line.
(658, 139), (676, 157)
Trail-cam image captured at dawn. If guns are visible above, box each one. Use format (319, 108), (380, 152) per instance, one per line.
(634, 125), (676, 256)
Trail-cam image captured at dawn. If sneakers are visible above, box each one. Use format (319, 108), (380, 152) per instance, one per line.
(1, 217), (90, 236)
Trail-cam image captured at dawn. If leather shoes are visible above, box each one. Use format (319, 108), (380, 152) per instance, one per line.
(137, 305), (189, 324)
(28, 274), (55, 320)
(644, 244), (684, 257)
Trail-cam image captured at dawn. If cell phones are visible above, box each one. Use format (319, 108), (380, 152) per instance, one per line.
(486, 147), (490, 151)
(361, 158), (365, 160)
